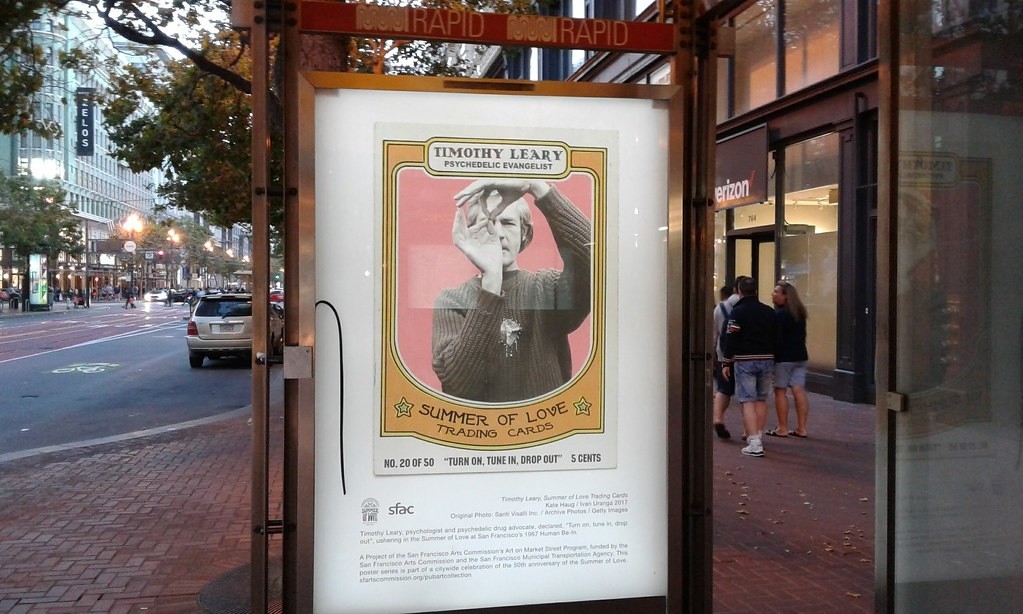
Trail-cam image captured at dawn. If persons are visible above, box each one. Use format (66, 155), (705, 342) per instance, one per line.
(9, 285), (19, 300)
(714, 275), (749, 440)
(167, 289), (172, 307)
(719, 286), (733, 300)
(53, 287), (60, 304)
(205, 286), (211, 294)
(114, 284), (121, 301)
(429, 175), (593, 404)
(190, 287), (197, 303)
(124, 289), (136, 310)
(68, 285), (74, 299)
(722, 278), (773, 456)
(98, 284), (113, 301)
(766, 278), (811, 438)
(182, 288), (190, 306)
(216, 286), (245, 294)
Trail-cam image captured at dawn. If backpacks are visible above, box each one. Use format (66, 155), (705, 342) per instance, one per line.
(720, 302), (729, 352)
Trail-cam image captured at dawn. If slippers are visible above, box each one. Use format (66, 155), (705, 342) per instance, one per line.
(788, 430), (808, 438)
(766, 427), (788, 437)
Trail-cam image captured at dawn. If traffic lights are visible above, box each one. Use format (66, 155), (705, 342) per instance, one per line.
(158, 250), (164, 259)
(92, 275), (99, 289)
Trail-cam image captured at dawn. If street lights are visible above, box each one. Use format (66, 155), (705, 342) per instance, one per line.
(167, 228), (181, 288)
(121, 213), (143, 298)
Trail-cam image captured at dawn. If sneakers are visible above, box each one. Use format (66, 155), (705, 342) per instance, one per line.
(741, 440), (764, 456)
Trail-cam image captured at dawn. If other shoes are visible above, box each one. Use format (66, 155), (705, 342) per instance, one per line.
(714, 422), (730, 439)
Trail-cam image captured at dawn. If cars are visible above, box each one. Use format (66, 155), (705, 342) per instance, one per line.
(144, 287), (245, 302)
(270, 290), (285, 302)
(184, 293), (284, 369)
